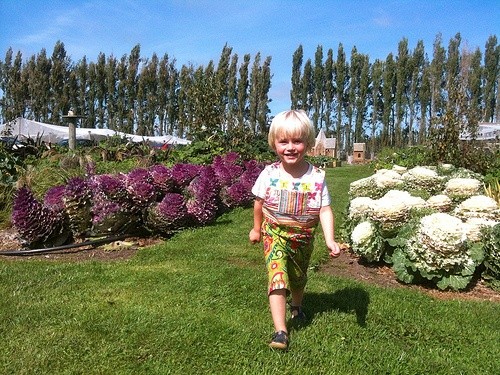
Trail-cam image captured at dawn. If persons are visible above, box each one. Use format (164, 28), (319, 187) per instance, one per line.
(248, 110), (340, 348)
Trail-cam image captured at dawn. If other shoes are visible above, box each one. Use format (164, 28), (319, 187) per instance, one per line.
(271, 330), (289, 348)
(288, 305), (306, 328)
